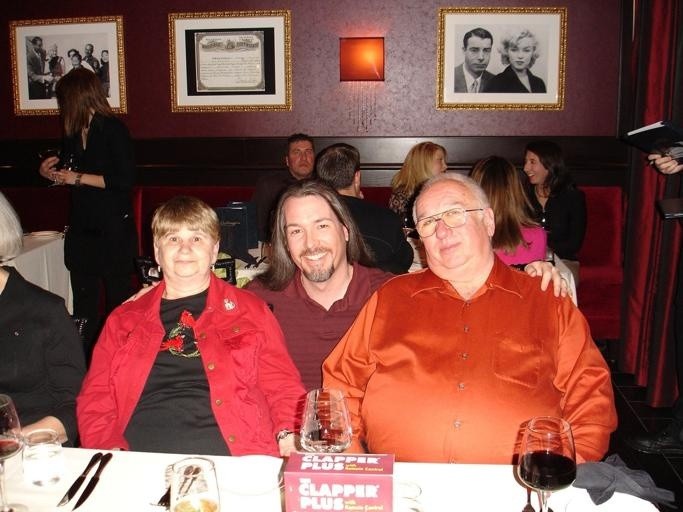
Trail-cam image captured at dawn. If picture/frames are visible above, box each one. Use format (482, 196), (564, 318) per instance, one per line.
(169, 11), (291, 112)
(9, 15), (127, 115)
(439, 6), (567, 110)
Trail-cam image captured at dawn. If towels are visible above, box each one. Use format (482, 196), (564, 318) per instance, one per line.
(574, 454), (673, 506)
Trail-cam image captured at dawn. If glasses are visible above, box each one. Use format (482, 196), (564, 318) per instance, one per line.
(402, 206), (491, 238)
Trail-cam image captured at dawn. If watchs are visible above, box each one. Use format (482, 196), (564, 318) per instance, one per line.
(275, 428), (293, 443)
(75, 172), (83, 185)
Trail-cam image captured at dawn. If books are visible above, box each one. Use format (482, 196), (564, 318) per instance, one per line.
(626, 121), (680, 155)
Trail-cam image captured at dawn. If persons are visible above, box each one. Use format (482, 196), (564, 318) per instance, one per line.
(82, 44), (100, 72)
(39, 67), (144, 371)
(27, 36), (53, 100)
(96, 50), (110, 98)
(521, 135), (588, 284)
(0, 187), (85, 448)
(455, 29), (496, 93)
(322, 174), (617, 464)
(250, 134), (315, 242)
(466, 156), (547, 271)
(315, 143), (414, 276)
(242, 182), (574, 393)
(389, 141), (447, 238)
(65, 50), (95, 74)
(44, 43), (66, 99)
(71, 54), (84, 70)
(647, 137), (682, 174)
(497, 30), (547, 94)
(77, 195), (307, 460)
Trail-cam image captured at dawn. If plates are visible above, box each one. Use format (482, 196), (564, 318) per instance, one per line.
(29, 230), (58, 238)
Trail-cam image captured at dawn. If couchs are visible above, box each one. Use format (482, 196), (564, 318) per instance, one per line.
(134, 186), (623, 359)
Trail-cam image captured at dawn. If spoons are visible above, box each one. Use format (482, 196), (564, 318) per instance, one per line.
(176, 465), (195, 497)
(184, 466), (202, 495)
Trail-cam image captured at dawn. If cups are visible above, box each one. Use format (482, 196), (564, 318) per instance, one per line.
(516, 415), (578, 512)
(36, 134), (66, 188)
(63, 154), (79, 172)
(168, 457), (222, 512)
(0, 393), (31, 511)
(299, 387), (354, 455)
(21, 427), (64, 491)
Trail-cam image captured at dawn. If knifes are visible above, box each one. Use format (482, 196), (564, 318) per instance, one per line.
(71, 453), (114, 512)
(245, 256), (259, 270)
(55, 452), (105, 509)
(253, 256), (268, 269)
